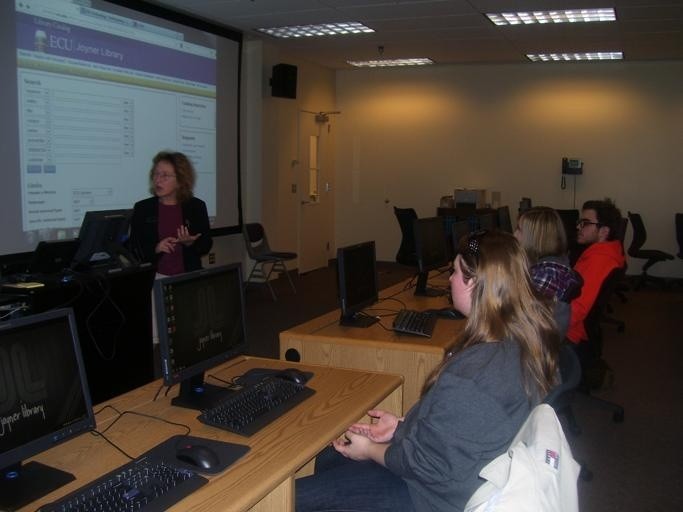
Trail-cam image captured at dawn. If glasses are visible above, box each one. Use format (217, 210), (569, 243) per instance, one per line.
(576, 217), (602, 228)
(152, 171), (177, 180)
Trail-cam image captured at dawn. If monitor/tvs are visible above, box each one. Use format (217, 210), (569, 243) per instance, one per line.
(27, 238), (82, 276)
(156, 261), (251, 412)
(407, 204), (513, 297)
(337, 240), (380, 328)
(0, 306), (97, 511)
(77, 208), (137, 275)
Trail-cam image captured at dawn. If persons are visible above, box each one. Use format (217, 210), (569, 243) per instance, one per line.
(296, 228), (563, 512)
(513, 206), (575, 308)
(128, 151), (213, 385)
(563, 196), (626, 349)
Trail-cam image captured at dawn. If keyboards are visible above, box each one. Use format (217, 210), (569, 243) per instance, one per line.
(34, 453), (208, 512)
(197, 376), (316, 438)
(391, 308), (437, 338)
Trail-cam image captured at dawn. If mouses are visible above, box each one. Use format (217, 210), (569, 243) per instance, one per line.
(437, 307), (462, 320)
(175, 441), (220, 474)
(276, 366), (309, 386)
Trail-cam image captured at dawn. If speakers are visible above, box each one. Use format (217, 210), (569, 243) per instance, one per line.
(270, 62), (299, 98)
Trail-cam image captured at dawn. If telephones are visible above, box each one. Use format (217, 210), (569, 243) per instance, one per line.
(562, 158), (582, 175)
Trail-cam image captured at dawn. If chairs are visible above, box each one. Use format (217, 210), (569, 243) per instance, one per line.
(668, 214), (683, 288)
(627, 211), (675, 292)
